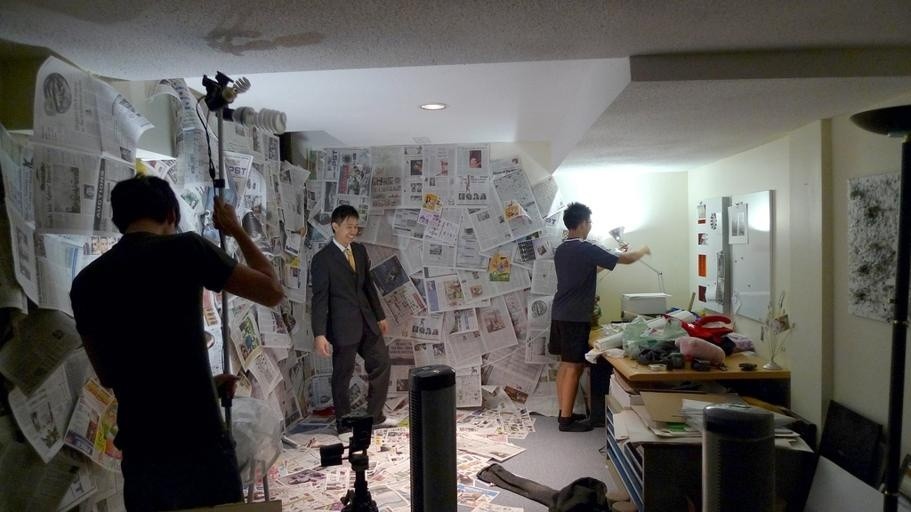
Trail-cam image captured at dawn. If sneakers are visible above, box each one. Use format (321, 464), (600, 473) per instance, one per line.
(558, 410), (591, 432)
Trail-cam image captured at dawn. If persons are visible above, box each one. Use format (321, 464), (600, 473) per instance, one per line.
(67, 173), (287, 512)
(548, 200), (652, 431)
(306, 204), (402, 435)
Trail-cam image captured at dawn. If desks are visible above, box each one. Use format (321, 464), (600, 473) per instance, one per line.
(587, 327), (791, 424)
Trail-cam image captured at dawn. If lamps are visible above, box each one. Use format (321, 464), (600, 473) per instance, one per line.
(609, 225), (666, 293)
(201, 71), (287, 135)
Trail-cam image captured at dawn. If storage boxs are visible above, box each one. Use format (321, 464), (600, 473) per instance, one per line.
(620, 296), (667, 315)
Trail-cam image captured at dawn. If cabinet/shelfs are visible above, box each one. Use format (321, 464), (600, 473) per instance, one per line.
(603, 394), (818, 512)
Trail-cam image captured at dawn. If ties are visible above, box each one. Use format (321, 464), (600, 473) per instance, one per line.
(345, 248), (355, 271)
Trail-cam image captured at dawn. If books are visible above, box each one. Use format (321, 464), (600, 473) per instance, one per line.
(608, 368), (817, 455)
